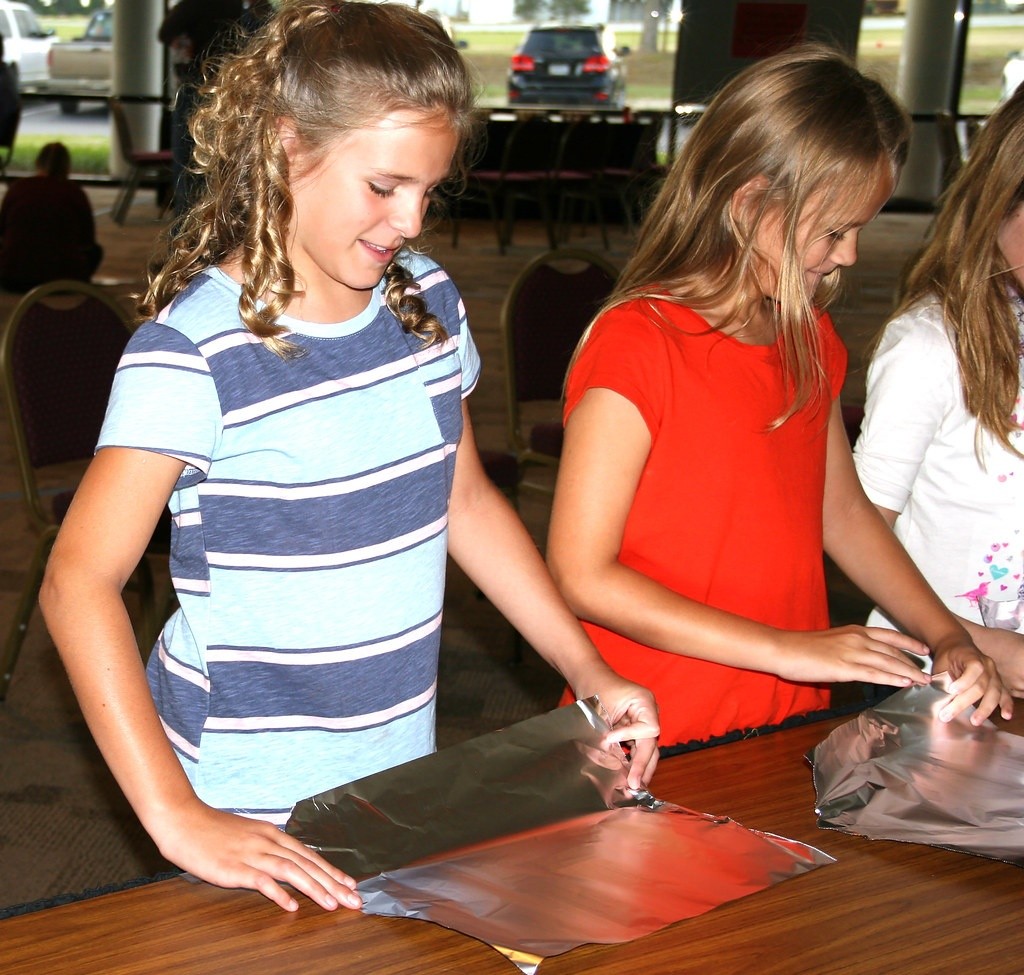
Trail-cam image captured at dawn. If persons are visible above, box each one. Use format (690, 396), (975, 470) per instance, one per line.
(0, 141), (103, 296)
(0, 34), (23, 163)
(35, 0), (663, 911)
(158, 0), (271, 219)
(545, 45), (1012, 755)
(851, 81), (1023, 701)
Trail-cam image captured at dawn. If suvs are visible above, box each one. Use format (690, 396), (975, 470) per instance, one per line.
(0, 0), (63, 93)
(506, 21), (632, 108)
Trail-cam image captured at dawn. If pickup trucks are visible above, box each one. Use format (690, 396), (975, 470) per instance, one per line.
(48, 10), (113, 113)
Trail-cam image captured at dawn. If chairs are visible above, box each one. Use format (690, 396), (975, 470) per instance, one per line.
(452, 107), (679, 255)
(107, 96), (173, 224)
(0, 282), (173, 711)
(503, 249), (619, 506)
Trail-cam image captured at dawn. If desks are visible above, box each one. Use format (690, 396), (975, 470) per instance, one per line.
(0, 712), (1024, 975)
